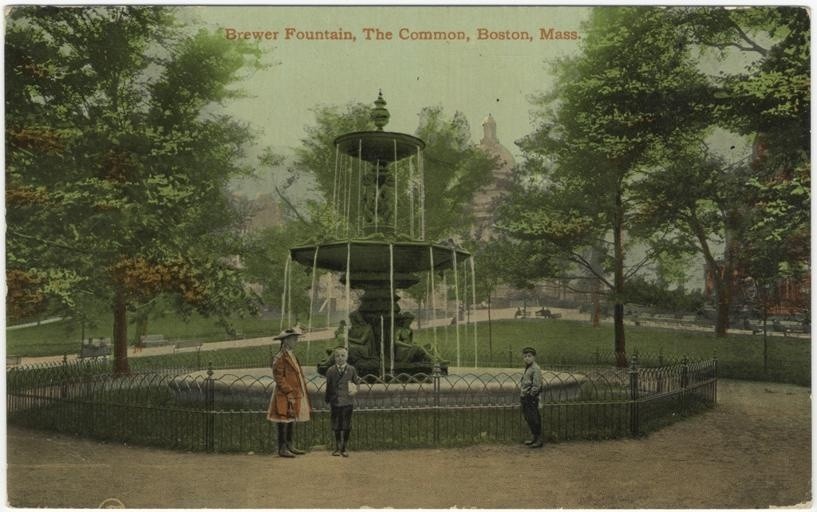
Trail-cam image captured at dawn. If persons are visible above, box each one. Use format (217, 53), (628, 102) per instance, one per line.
(266, 328), (312, 459)
(324, 346), (364, 457)
(517, 346), (544, 449)
(78, 336), (110, 360)
(514, 306), (551, 320)
(316, 311), (451, 368)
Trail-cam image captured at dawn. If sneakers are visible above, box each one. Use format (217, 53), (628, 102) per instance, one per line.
(332, 450), (341, 456)
(342, 451), (348, 457)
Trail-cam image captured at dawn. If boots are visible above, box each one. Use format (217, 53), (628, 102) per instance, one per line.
(277, 443), (296, 458)
(287, 441), (306, 455)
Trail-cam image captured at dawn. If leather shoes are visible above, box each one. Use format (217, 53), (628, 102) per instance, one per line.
(532, 440), (543, 447)
(524, 437), (534, 444)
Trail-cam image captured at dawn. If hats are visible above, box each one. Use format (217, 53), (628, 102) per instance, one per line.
(272, 327), (301, 341)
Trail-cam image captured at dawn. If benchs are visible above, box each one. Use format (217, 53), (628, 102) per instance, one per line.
(79, 337), (114, 348)
(79, 346), (112, 361)
(518, 309), (563, 320)
(172, 339), (203, 355)
(138, 333), (169, 351)
(6, 355), (22, 371)
